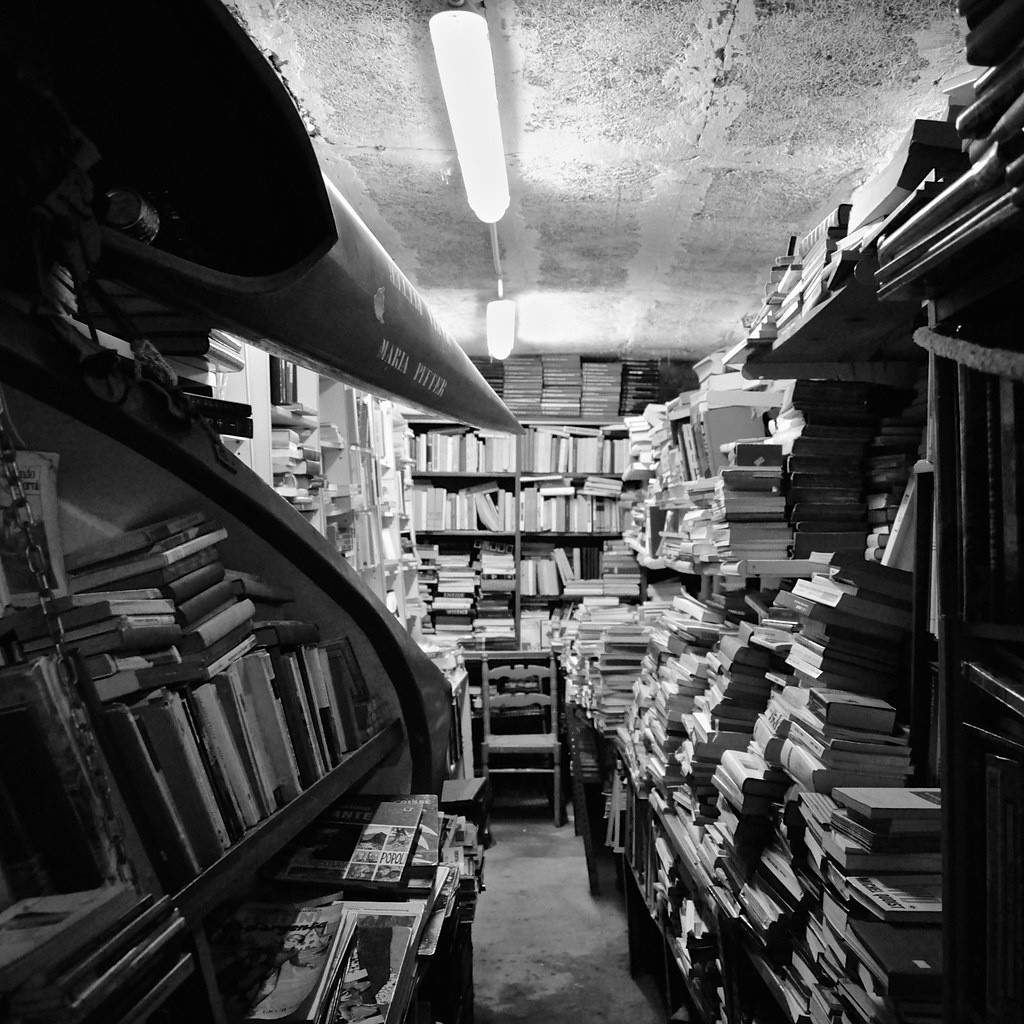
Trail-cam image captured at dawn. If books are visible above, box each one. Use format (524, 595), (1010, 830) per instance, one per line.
(0, 0), (1023, 1024)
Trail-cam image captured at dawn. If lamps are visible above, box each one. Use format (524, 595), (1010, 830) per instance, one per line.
(428, 0), (511, 224)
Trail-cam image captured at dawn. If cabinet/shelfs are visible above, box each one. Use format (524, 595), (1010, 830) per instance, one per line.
(149, 146), (1024, 1024)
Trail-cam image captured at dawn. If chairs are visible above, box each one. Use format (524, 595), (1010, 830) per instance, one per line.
(481, 652), (563, 825)
